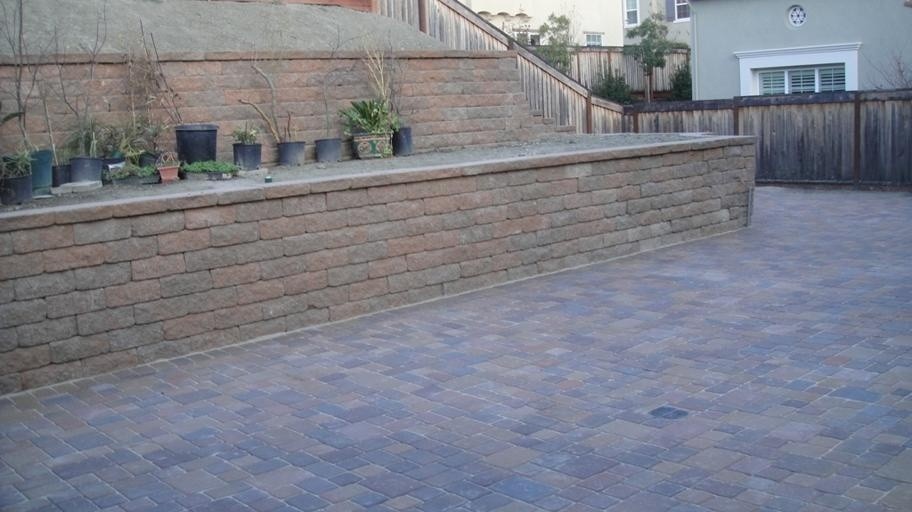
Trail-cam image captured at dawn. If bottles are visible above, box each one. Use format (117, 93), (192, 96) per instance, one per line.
(265, 172), (271, 184)
